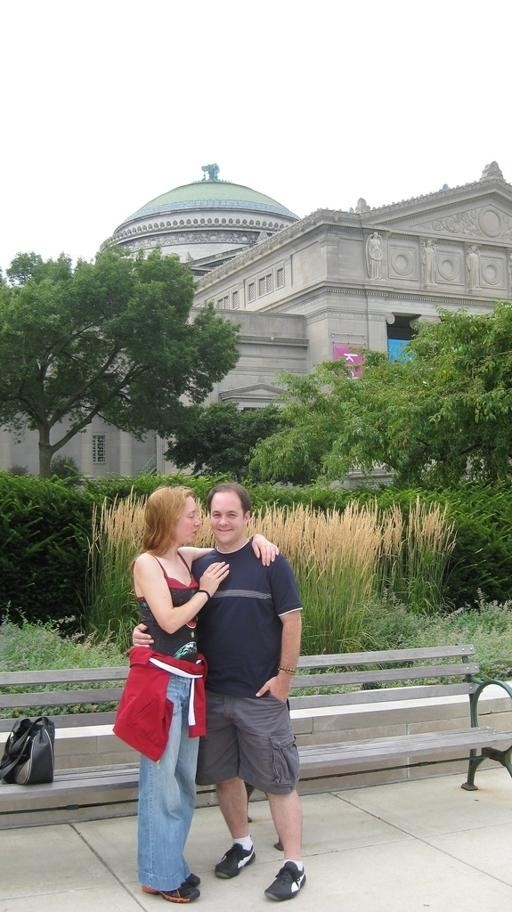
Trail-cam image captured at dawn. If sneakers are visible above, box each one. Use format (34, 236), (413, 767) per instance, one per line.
(214, 842), (256, 879)
(262, 860), (306, 901)
(186, 873), (201, 887)
(141, 879), (201, 903)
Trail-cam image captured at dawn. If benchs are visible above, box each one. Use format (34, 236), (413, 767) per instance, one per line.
(1, 642), (512, 818)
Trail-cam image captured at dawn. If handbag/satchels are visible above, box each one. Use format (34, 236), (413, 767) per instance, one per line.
(0, 717), (56, 785)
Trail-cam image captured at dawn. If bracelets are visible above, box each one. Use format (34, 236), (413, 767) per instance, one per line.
(197, 588), (212, 603)
(277, 666), (295, 673)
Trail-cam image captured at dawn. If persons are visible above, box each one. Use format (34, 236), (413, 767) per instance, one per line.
(110, 486), (279, 904)
(130, 482), (311, 902)
